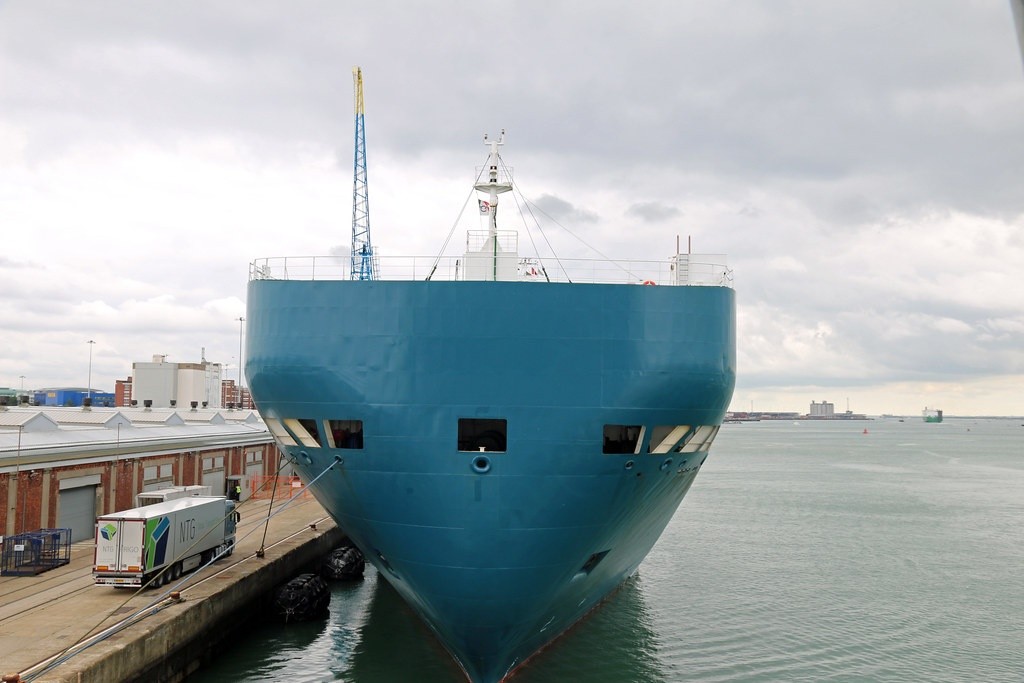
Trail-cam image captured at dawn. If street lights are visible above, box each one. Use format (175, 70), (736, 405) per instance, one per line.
(17, 426), (24, 479)
(87, 340), (96, 398)
(19, 375), (26, 400)
(236, 316), (245, 402)
(117, 423), (123, 465)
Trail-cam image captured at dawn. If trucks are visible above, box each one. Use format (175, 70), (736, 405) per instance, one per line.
(136, 486), (211, 508)
(91, 495), (240, 589)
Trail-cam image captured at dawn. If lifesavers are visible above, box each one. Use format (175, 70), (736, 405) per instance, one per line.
(644, 281), (655, 285)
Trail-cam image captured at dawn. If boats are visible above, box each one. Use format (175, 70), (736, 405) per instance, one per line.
(922, 407), (943, 422)
(244, 65), (737, 683)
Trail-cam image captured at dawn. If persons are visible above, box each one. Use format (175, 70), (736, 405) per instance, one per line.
(232, 483), (243, 503)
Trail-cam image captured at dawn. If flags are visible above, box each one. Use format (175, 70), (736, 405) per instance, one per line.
(478, 199), (490, 216)
